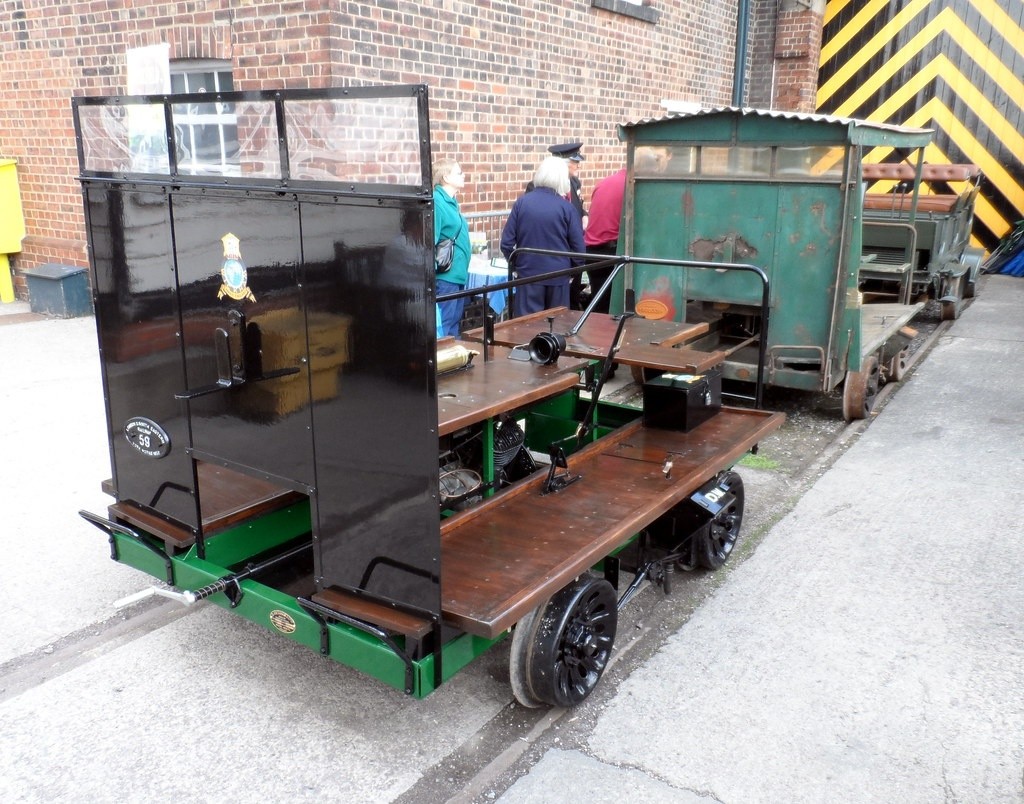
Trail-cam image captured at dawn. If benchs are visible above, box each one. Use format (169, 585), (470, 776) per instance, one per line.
(834, 162), (983, 254)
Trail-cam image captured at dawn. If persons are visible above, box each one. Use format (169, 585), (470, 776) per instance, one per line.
(582, 145), (657, 313)
(500, 156), (586, 317)
(431, 157), (471, 339)
(524, 142), (589, 311)
(653, 145), (673, 174)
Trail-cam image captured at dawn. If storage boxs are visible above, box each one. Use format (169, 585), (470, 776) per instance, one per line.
(640, 370), (724, 432)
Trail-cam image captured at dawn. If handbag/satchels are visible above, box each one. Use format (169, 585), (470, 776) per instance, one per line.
(434, 239), (454, 273)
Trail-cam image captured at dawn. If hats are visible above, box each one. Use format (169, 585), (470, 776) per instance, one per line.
(548, 142), (584, 161)
